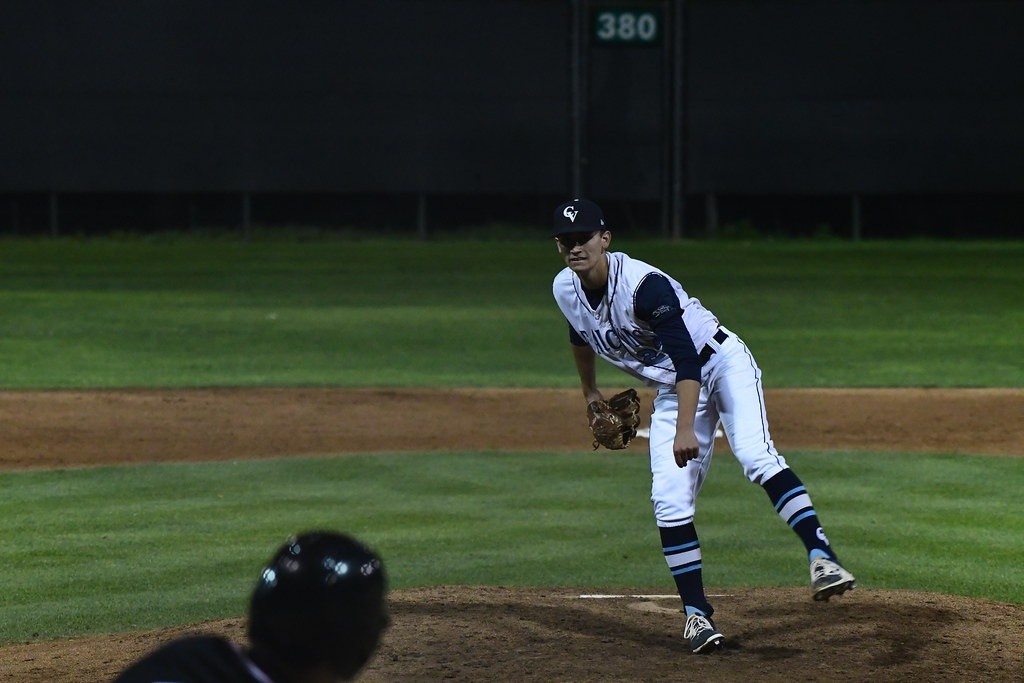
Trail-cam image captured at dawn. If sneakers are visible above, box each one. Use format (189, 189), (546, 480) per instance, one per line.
(684, 613), (725, 651)
(809, 557), (856, 601)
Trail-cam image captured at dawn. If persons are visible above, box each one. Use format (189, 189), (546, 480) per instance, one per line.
(553, 200), (859, 653)
(113, 532), (390, 683)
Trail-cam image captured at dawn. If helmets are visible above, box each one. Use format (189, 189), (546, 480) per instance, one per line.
(252, 531), (388, 677)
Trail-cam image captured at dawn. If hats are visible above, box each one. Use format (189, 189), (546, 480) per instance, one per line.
(549, 197), (607, 238)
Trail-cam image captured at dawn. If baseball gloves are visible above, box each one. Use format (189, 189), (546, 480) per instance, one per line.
(585, 389), (641, 450)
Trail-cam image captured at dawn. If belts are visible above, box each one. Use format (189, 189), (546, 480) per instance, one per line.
(699, 330), (728, 368)
